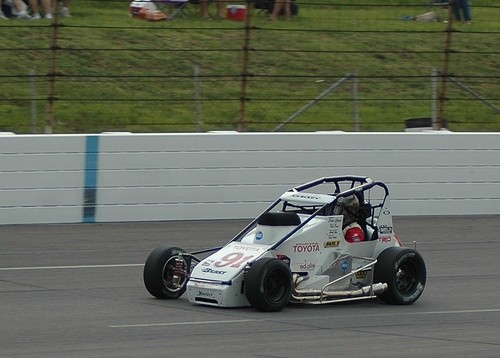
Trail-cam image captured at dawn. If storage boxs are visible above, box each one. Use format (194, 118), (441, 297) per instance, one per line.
(226, 5), (247, 20)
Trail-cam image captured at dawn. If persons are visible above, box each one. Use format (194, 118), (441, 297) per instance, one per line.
(0, 0), (72, 20)
(199, 0), (226, 21)
(266, 0), (292, 21)
(453, 0), (472, 24)
(333, 194), (365, 242)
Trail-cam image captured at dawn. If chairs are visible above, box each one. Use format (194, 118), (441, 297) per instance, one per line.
(366, 223), (376, 240)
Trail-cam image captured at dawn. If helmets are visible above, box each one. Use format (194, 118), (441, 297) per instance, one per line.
(333, 194), (359, 228)
